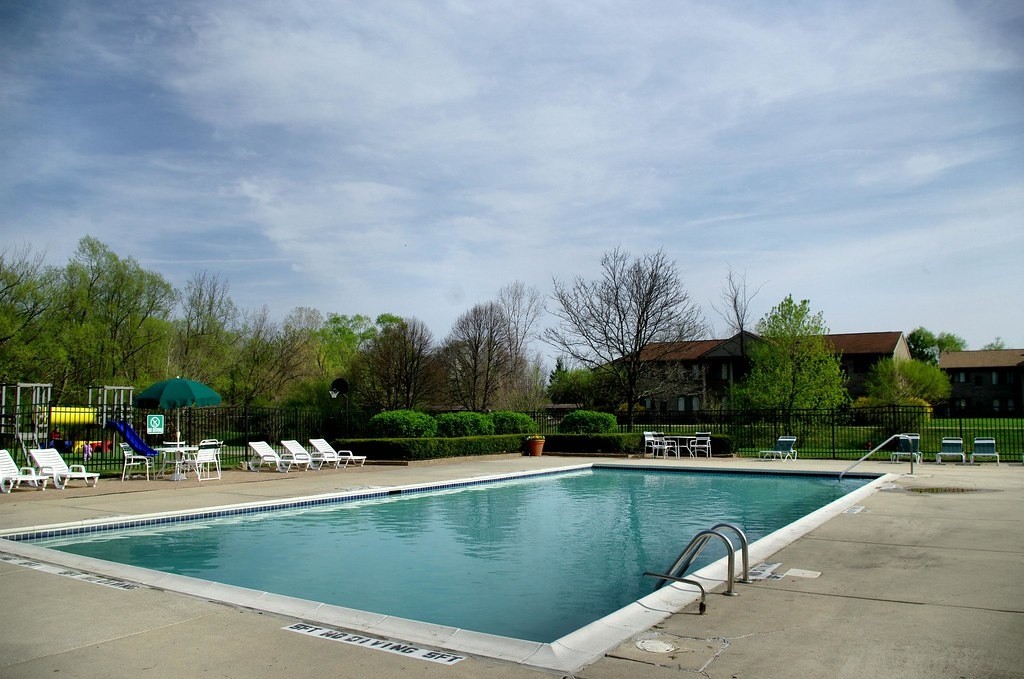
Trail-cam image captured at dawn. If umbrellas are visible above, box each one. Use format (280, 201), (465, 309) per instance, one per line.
(132, 376), (222, 481)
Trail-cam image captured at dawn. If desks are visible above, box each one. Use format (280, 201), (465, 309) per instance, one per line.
(154, 447), (198, 480)
(664, 436), (696, 459)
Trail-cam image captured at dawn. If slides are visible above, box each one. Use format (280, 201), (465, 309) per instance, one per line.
(108, 419), (159, 456)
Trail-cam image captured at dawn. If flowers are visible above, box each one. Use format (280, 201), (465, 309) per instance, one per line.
(526, 435), (545, 440)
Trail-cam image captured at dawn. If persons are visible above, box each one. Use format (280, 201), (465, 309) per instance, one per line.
(83, 441), (92, 461)
(50, 427), (63, 448)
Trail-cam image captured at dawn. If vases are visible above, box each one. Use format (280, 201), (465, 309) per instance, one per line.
(530, 440), (545, 456)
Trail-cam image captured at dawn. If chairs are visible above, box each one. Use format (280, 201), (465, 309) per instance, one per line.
(652, 433), (669, 459)
(0, 449), (49, 493)
(188, 439), (224, 482)
(118, 443), (155, 481)
(759, 436), (797, 462)
(936, 437), (966, 464)
(162, 441), (186, 478)
(890, 433), (922, 464)
(29, 448), (100, 490)
(690, 432), (711, 458)
(970, 437), (999, 466)
(248, 441), (310, 473)
(310, 438), (366, 470)
(280, 440), (341, 470)
(643, 431), (657, 454)
